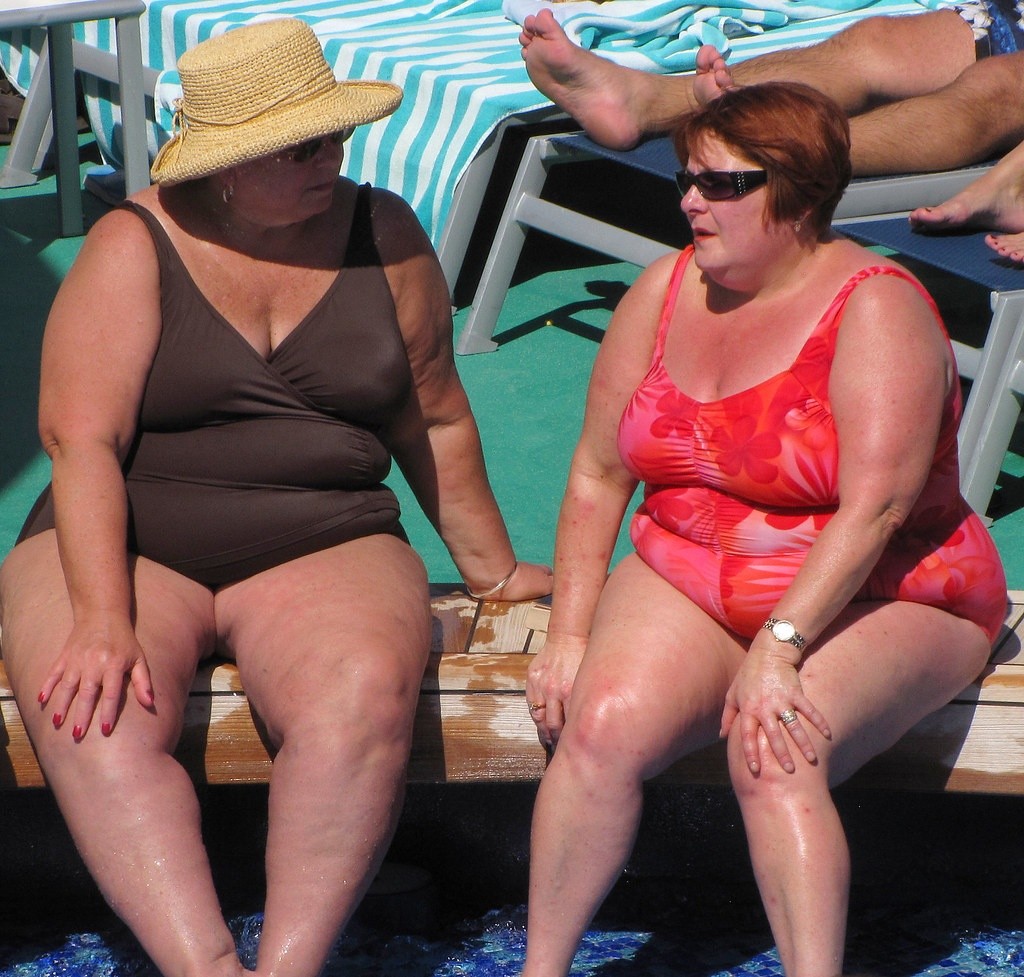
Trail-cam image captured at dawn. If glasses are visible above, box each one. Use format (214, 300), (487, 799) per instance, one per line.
(674, 166), (767, 202)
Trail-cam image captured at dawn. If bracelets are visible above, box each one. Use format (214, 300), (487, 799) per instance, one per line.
(466, 564), (513, 599)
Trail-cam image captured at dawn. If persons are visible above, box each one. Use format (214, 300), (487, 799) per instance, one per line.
(515, 1), (1024, 171)
(2, 18), (552, 977)
(909, 139), (1024, 269)
(515, 79), (1010, 977)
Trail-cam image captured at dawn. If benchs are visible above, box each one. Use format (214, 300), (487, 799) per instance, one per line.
(0, 580), (1024, 794)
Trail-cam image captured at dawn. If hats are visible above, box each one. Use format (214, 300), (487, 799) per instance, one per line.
(148, 18), (404, 190)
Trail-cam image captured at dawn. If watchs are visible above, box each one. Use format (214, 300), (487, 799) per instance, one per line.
(761, 617), (808, 653)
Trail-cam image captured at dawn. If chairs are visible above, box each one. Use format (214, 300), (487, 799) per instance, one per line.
(0, 0), (1024, 529)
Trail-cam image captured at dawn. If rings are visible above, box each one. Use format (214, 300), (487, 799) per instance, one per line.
(529, 703), (545, 712)
(781, 709), (797, 726)
